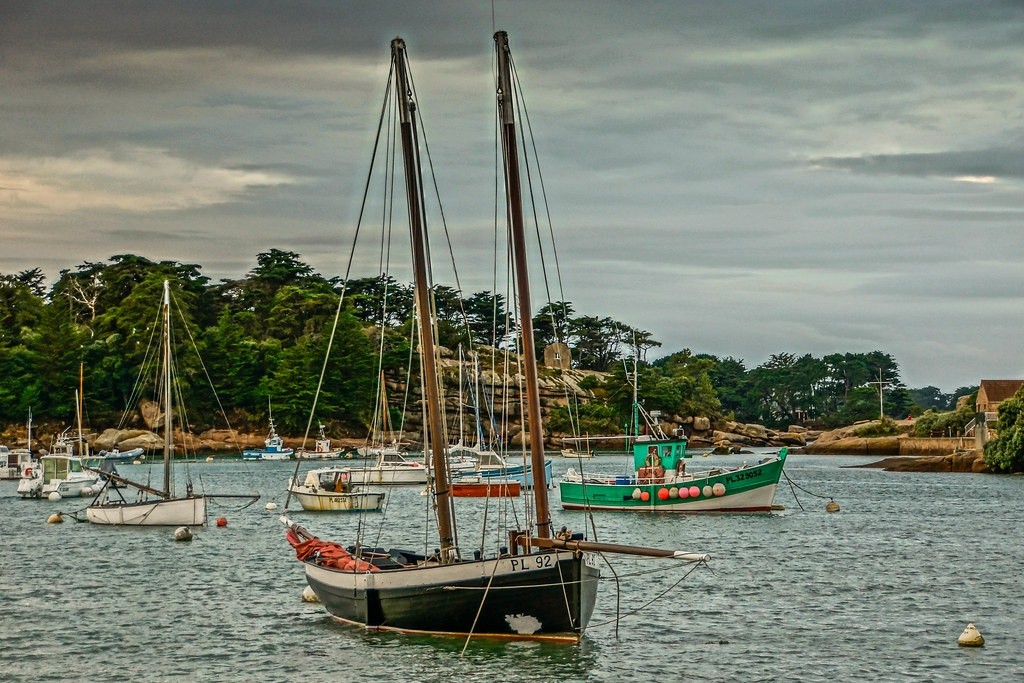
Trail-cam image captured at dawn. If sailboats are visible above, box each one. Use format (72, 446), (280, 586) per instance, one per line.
(81, 280), (261, 525)
(281, 0), (711, 643)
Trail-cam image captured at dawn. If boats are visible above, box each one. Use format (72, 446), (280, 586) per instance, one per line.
(295, 343), (552, 497)
(561, 449), (594, 458)
(95, 445), (144, 465)
(560, 321), (788, 512)
(242, 394), (294, 461)
(288, 465), (386, 514)
(0, 406), (129, 498)
